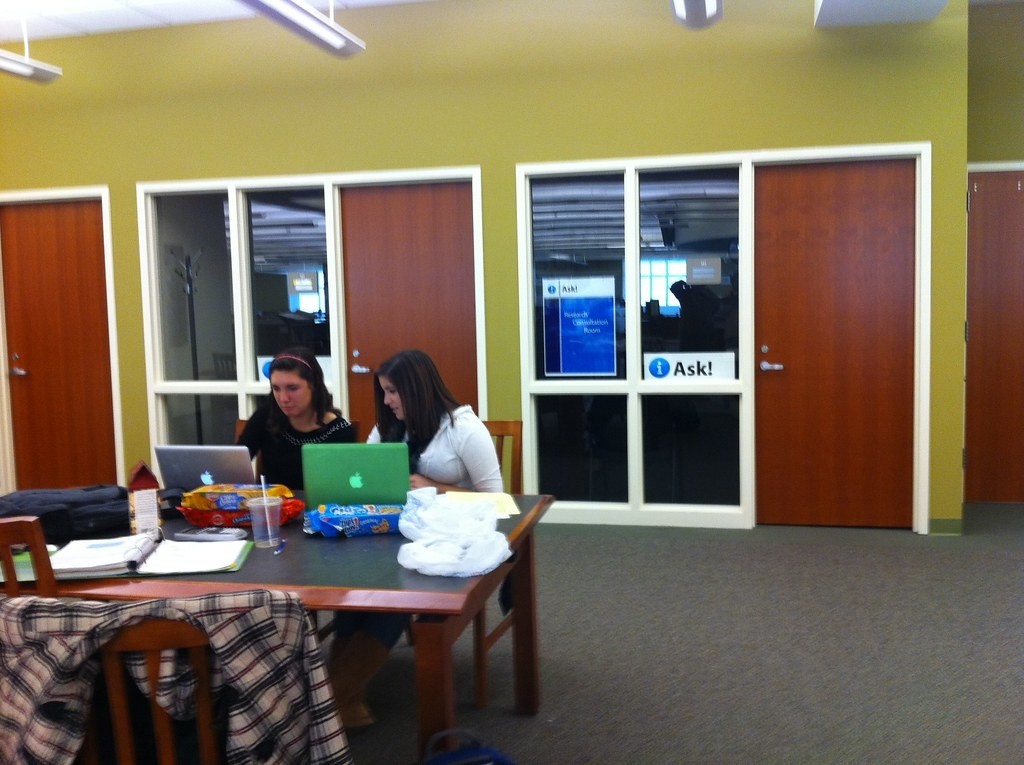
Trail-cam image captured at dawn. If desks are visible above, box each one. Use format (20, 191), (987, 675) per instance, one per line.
(0, 491), (556, 764)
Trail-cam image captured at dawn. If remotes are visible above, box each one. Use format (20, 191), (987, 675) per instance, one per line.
(174, 527), (248, 541)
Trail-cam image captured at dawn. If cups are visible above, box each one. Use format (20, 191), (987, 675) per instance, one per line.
(246, 497), (281, 548)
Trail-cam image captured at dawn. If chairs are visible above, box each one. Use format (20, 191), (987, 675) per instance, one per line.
(80, 615), (232, 765)
(0, 515), (59, 598)
(480, 420), (524, 710)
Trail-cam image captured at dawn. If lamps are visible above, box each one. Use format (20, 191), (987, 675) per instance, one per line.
(0, 0), (63, 84)
(235, 0), (367, 59)
(669, 0), (723, 31)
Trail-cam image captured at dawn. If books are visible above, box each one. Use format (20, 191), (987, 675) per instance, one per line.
(44, 526), (253, 578)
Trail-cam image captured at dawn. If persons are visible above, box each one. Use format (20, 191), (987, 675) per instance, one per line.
(234, 346), (356, 490)
(324, 350), (505, 729)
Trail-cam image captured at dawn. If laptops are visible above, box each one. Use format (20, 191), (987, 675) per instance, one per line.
(302, 444), (410, 513)
(156, 446), (255, 492)
